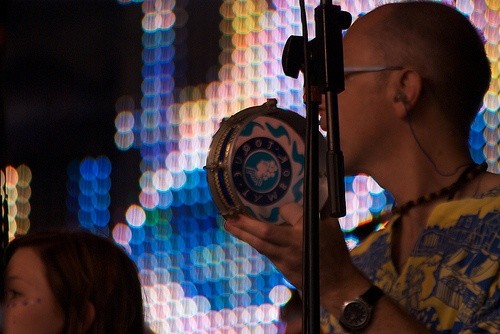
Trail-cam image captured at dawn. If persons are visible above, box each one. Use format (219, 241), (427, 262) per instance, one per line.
(224, 0), (500, 333)
(0, 225), (156, 334)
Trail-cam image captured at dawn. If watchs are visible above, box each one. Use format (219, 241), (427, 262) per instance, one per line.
(338, 284), (384, 334)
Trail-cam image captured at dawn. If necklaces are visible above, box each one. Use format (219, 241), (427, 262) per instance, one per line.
(390, 161), (489, 216)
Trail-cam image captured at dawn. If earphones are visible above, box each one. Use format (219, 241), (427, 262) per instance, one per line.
(396, 93), (405, 101)
